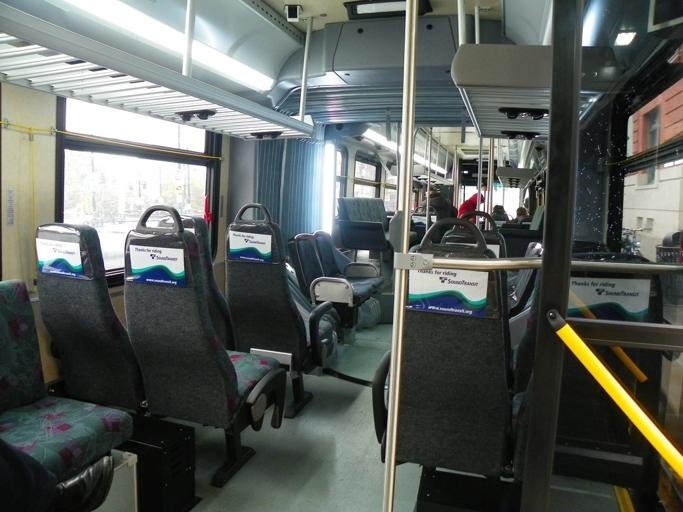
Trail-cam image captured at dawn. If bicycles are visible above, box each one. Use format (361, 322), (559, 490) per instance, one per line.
(621, 226), (653, 258)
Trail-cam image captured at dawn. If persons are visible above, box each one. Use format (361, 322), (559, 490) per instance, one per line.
(408, 185), (458, 250)
(511, 207), (527, 222)
(455, 192), (485, 229)
(489, 205), (508, 223)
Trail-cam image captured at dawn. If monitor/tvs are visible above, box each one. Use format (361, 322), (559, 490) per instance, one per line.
(460, 163), (488, 187)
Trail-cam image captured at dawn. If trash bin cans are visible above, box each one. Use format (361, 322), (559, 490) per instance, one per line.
(656, 231), (683, 305)
